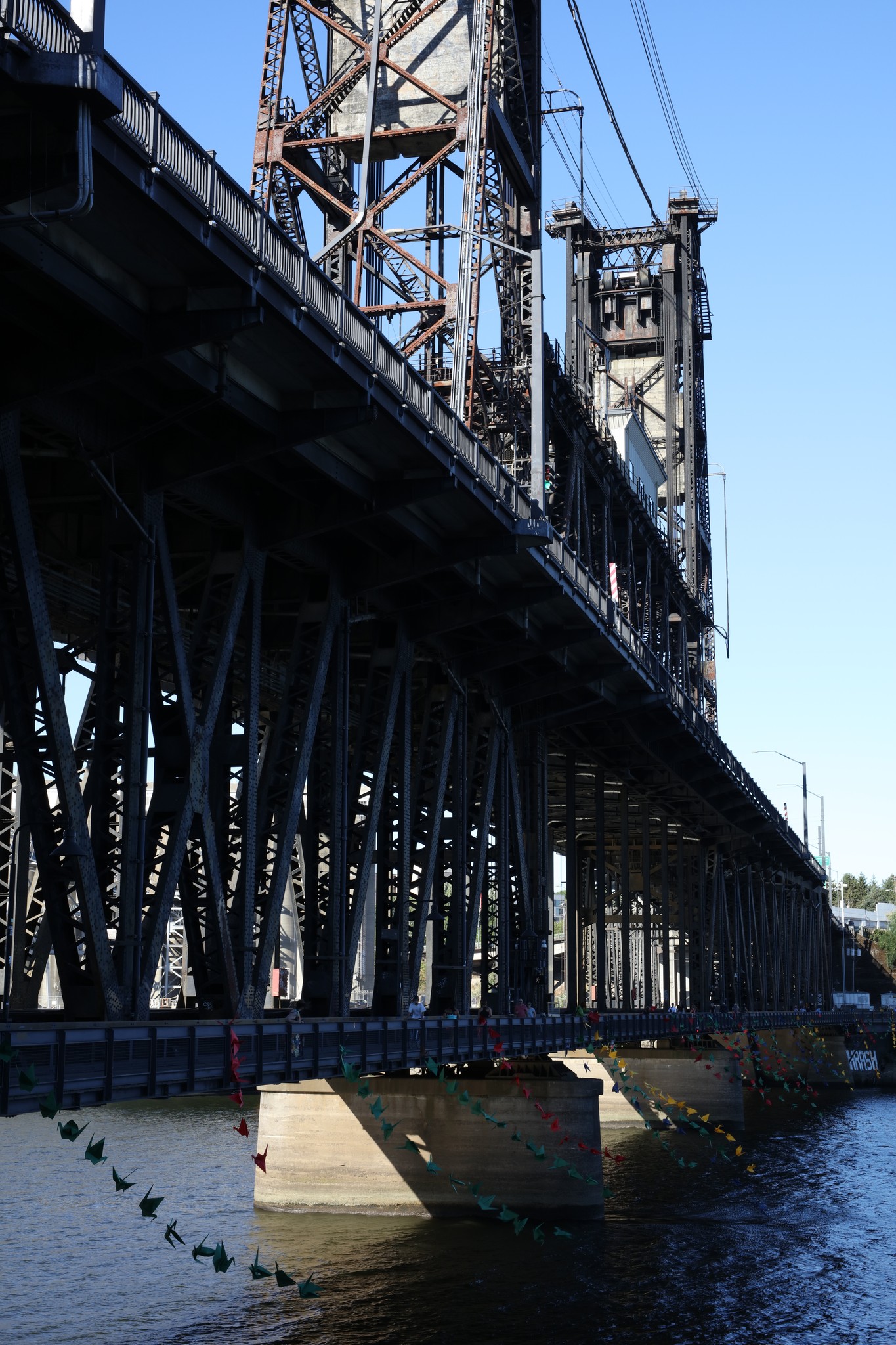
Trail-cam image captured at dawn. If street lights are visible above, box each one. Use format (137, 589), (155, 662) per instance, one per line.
(752, 750), (826, 871)
(826, 867), (840, 927)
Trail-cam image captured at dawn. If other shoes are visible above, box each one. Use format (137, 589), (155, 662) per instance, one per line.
(448, 1043), (454, 1047)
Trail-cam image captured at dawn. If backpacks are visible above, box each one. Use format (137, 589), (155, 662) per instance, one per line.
(570, 1007), (580, 1022)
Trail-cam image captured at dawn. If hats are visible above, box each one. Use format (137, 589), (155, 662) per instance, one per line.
(413, 995), (419, 999)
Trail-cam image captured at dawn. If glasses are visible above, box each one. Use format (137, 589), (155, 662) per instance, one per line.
(414, 999), (419, 1001)
(527, 1004), (531, 1005)
(519, 1001), (522, 1002)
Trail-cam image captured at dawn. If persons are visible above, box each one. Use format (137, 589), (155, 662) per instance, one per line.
(285, 1000), (305, 1059)
(831, 1007), (834, 1012)
(799, 1006), (806, 1012)
(667, 1003), (677, 1013)
(838, 1005), (896, 1019)
(676, 1001), (685, 1017)
(815, 1008), (823, 1015)
(732, 1004), (739, 1013)
(806, 1006), (812, 1012)
(404, 995), (426, 1041)
(719, 1003), (728, 1013)
(740, 1004), (749, 1013)
(525, 1002), (536, 1039)
(709, 1003), (716, 1013)
(794, 1006), (798, 1012)
(640, 1006), (652, 1014)
(443, 1001), (461, 1048)
(573, 1002), (594, 1037)
(515, 998), (529, 1018)
(478, 1000), (492, 1044)
(690, 1004), (697, 1014)
